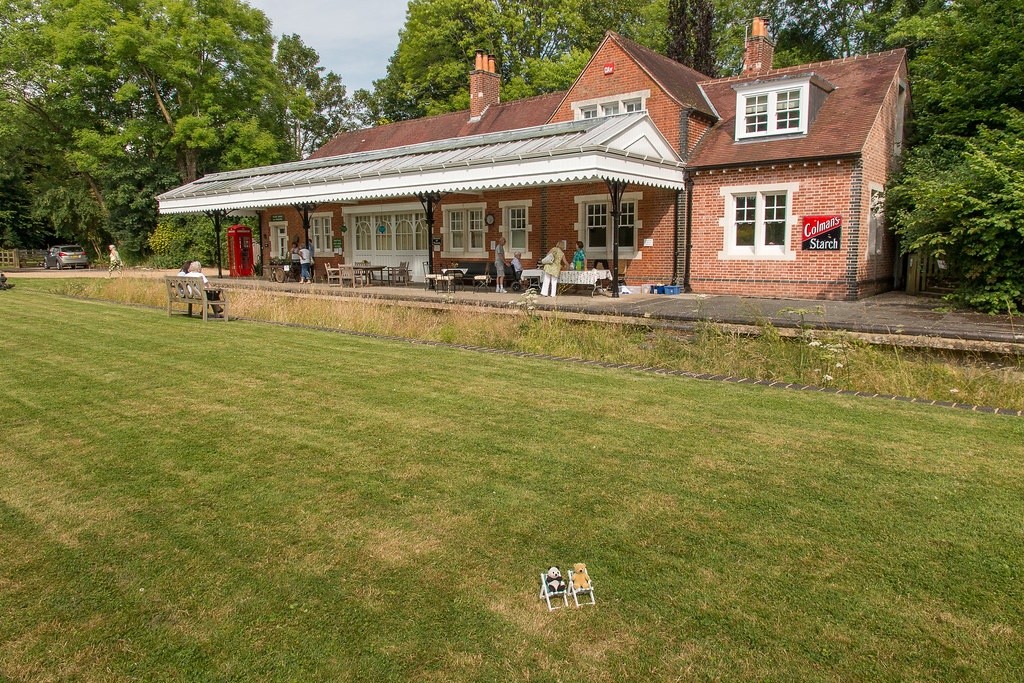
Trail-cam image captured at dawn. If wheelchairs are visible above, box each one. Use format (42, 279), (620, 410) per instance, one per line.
(506, 264), (523, 291)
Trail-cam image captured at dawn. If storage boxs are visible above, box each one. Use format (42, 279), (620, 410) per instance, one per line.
(618, 284), (680, 294)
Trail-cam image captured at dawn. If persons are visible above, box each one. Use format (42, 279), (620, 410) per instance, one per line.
(290, 242), (300, 282)
(570, 241), (588, 293)
(175, 260), (223, 318)
(494, 237), (511, 293)
(540, 241), (569, 297)
(309, 238), (315, 283)
(109, 244), (122, 275)
(298, 243), (312, 284)
(511, 251), (524, 279)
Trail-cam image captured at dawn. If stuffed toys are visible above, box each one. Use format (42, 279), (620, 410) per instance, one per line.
(571, 563), (590, 589)
(545, 566), (565, 591)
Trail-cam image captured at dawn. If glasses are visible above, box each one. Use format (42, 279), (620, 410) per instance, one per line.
(501, 240), (506, 243)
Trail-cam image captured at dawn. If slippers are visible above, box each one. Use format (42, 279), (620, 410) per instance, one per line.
(218, 306), (224, 314)
(214, 312), (225, 318)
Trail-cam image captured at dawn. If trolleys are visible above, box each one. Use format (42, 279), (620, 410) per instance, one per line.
(268, 260), (300, 283)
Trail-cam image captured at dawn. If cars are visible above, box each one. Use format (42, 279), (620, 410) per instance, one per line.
(43, 245), (87, 270)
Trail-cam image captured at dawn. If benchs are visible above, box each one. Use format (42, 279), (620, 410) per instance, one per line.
(163, 274), (230, 323)
(454, 262), (515, 288)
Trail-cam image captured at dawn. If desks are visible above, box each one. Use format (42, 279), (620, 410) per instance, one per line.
(520, 269), (613, 297)
(352, 264), (387, 286)
(442, 268), (468, 293)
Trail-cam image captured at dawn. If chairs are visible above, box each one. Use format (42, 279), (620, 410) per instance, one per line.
(325, 261), (414, 288)
(422, 261), (493, 294)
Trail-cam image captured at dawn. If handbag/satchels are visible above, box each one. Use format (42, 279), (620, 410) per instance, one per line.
(309, 256), (315, 267)
(541, 251), (557, 264)
(576, 260), (582, 271)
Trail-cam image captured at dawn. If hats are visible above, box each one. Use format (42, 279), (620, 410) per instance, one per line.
(292, 241), (298, 248)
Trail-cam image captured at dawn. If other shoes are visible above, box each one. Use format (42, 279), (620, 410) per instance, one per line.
(500, 288), (508, 293)
(496, 288), (500, 293)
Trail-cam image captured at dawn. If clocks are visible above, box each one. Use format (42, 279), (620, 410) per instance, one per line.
(485, 212), (495, 226)
